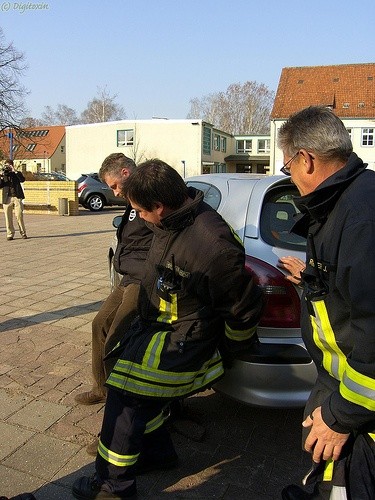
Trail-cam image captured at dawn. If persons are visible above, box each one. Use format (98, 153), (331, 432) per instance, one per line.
(0, 158), (27, 239)
(277, 105), (375, 500)
(72, 158), (261, 500)
(74, 153), (154, 456)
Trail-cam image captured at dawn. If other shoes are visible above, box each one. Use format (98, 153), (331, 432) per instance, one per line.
(75, 391), (106, 405)
(7, 237), (13, 240)
(86, 439), (99, 456)
(281, 484), (313, 500)
(20, 232), (27, 239)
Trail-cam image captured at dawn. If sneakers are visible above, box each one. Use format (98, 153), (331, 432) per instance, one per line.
(72, 472), (138, 500)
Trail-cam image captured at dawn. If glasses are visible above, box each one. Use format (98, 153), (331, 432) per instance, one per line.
(280, 151), (314, 176)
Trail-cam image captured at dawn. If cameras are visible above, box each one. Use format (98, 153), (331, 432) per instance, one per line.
(2, 164), (11, 175)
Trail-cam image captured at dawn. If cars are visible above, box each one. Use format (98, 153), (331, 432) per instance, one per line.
(181, 171), (319, 410)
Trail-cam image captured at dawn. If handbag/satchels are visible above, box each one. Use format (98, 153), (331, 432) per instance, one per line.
(0, 186), (9, 204)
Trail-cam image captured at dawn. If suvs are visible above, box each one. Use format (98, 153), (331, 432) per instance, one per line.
(76, 173), (128, 211)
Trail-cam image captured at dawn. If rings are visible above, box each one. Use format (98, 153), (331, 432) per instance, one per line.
(310, 412), (313, 420)
